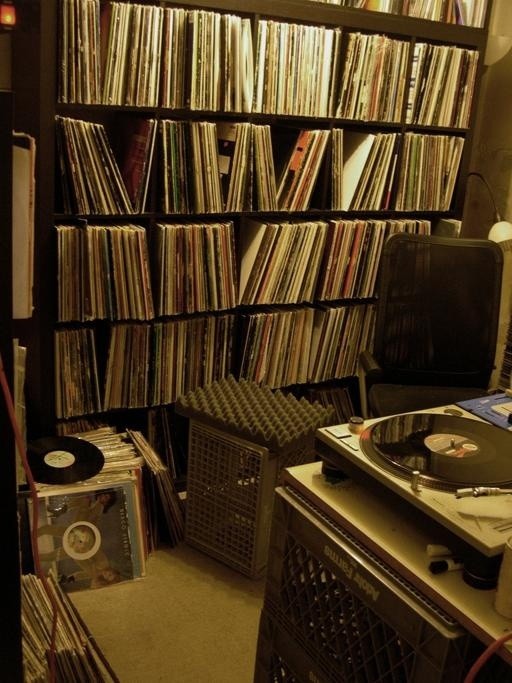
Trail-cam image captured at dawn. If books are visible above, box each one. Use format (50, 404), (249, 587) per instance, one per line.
(52, 113), (466, 219)
(58, 0), (482, 134)
(51, 301), (378, 429)
(54, 216), (463, 326)
(29, 425), (187, 595)
(21, 570), (122, 682)
(13, 131), (37, 572)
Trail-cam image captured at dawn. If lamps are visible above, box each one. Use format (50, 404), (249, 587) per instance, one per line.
(457, 171), (512, 244)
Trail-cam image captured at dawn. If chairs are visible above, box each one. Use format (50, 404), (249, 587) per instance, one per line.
(359, 231), (504, 423)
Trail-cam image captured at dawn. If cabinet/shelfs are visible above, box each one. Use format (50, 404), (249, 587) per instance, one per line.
(13, 1), (492, 512)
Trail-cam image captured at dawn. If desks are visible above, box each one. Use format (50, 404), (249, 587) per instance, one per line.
(252, 393), (512, 681)
